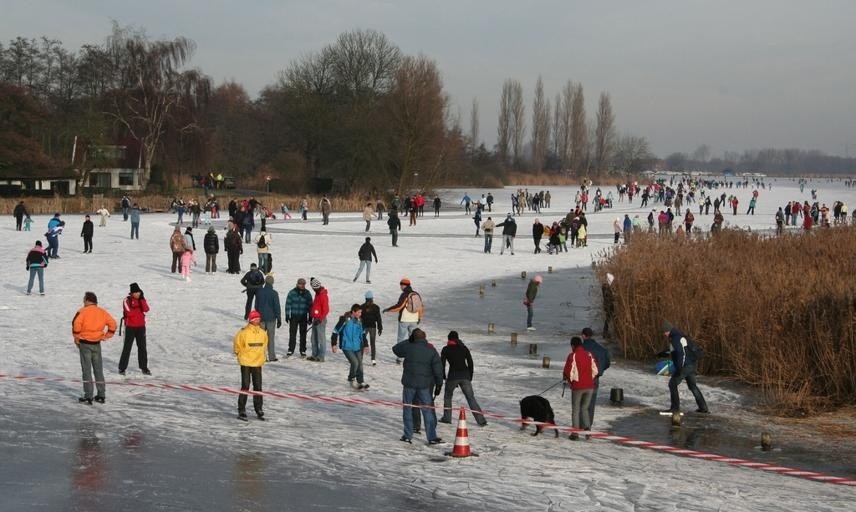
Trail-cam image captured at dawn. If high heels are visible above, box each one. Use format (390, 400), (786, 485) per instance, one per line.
(444, 408), (479, 458)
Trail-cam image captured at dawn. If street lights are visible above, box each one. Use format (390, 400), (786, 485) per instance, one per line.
(671, 344), (708, 372)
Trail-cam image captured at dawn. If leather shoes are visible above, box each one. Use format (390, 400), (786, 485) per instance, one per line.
(655, 350), (670, 358)
(139, 290), (143, 300)
(277, 314), (321, 328)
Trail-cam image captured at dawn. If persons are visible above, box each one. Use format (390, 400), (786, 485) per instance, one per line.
(392, 328), (486, 445)
(602, 272), (616, 339)
(562, 327), (611, 439)
(15, 201), (65, 295)
(241, 263), (266, 321)
(459, 173), (855, 255)
(363, 193), (441, 231)
(383, 278), (423, 363)
(234, 310), (268, 420)
(72, 292), (117, 404)
(353, 237), (378, 283)
(525, 274), (542, 330)
(654, 320), (709, 414)
(170, 172), (331, 281)
(388, 212), (401, 247)
(331, 290), (383, 390)
(81, 195), (140, 253)
(257, 275), (329, 361)
(118, 283), (151, 375)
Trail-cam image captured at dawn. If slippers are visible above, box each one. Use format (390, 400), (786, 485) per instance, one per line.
(258, 231), (267, 248)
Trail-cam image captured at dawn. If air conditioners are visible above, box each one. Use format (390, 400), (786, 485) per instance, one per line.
(518, 394), (560, 439)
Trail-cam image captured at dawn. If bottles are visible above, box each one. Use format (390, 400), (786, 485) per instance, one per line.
(400, 279), (410, 285)
(310, 277), (322, 289)
(129, 283), (141, 293)
(297, 278), (306, 284)
(248, 310), (261, 323)
(365, 290), (374, 299)
(535, 276), (543, 283)
(661, 319), (674, 332)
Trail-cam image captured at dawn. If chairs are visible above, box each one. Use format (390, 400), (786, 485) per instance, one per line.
(399, 417), (488, 444)
(526, 326), (537, 330)
(269, 350), (324, 362)
(659, 409), (710, 417)
(237, 412), (248, 420)
(119, 367), (126, 376)
(584, 434), (593, 442)
(569, 432), (580, 441)
(141, 367), (150, 375)
(350, 356), (405, 388)
(256, 410), (265, 417)
(77, 395), (106, 405)
